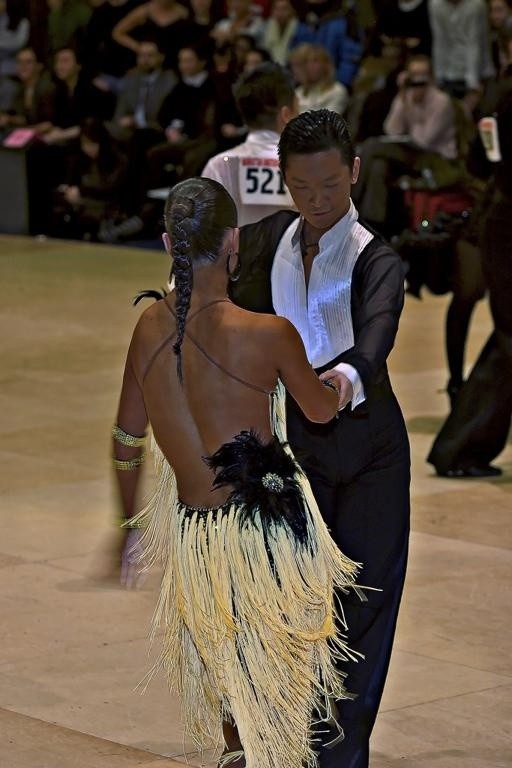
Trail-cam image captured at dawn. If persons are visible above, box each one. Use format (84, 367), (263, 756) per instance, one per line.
(0, 0), (511, 243)
(84, 172), (380, 768)
(426, 13), (512, 487)
(225, 101), (414, 766)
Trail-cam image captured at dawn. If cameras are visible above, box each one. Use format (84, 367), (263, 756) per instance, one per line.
(406, 75), (427, 86)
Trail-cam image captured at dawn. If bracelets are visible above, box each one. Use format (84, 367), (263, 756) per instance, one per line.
(321, 377), (343, 420)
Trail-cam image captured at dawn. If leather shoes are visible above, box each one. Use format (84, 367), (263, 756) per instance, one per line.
(438, 466), (502, 477)
